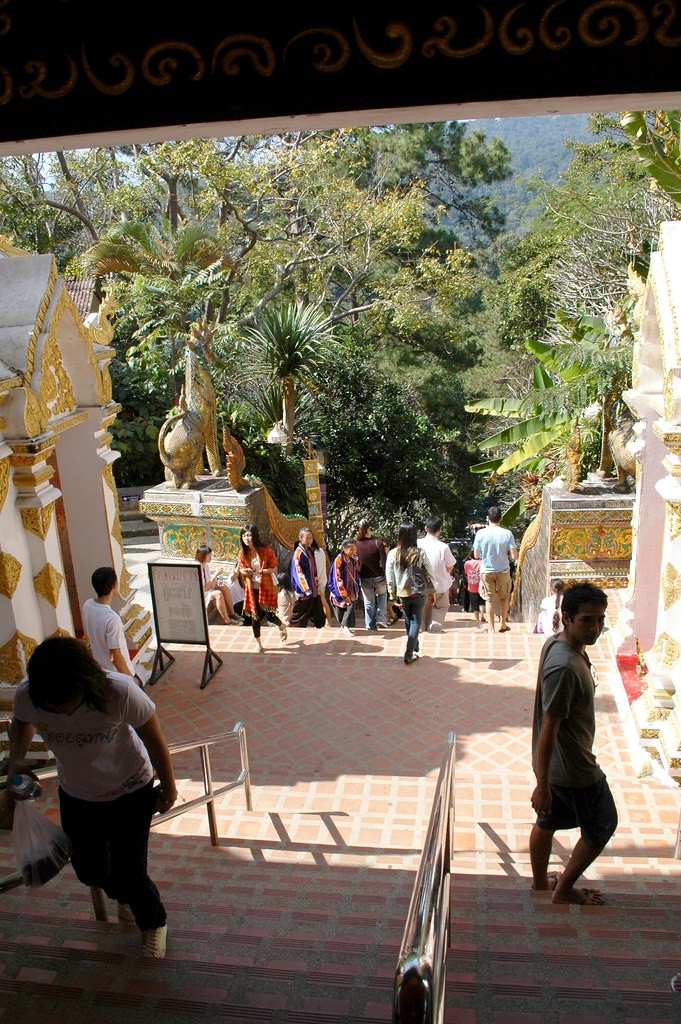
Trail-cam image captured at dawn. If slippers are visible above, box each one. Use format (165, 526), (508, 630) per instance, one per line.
(499, 626), (511, 632)
(483, 628), (489, 633)
(552, 889), (604, 907)
(534, 876), (560, 891)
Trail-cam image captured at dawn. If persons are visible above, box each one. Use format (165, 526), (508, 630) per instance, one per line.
(534, 581), (565, 637)
(6, 637), (178, 959)
(238, 524), (288, 654)
(473, 507), (518, 635)
(194, 516), (457, 633)
(528, 583), (619, 906)
(80, 566), (145, 693)
(385, 520), (437, 665)
(460, 550), (487, 623)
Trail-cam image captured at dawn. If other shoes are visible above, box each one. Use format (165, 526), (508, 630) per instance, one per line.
(279, 624), (287, 641)
(481, 619), (488, 623)
(415, 640), (419, 652)
(229, 613), (245, 621)
(405, 654), (418, 664)
(257, 647), (264, 652)
(341, 626), (356, 637)
(227, 619), (243, 626)
(376, 621), (387, 628)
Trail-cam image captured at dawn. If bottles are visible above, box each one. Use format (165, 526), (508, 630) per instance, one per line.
(11, 774), (42, 800)
(217, 574), (224, 586)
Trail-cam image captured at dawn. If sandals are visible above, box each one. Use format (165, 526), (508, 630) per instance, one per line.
(387, 613), (398, 626)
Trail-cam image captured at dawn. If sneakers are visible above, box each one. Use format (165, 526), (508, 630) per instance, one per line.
(117, 903), (136, 926)
(138, 923), (167, 958)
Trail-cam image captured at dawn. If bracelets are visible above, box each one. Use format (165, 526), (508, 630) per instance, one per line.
(159, 789), (175, 804)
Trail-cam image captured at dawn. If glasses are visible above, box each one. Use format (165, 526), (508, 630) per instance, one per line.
(590, 664), (599, 687)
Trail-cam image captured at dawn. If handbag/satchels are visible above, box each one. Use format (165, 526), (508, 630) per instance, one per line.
(11, 796), (70, 890)
(410, 548), (436, 595)
(375, 540), (386, 572)
(270, 572), (279, 586)
(354, 582), (361, 601)
